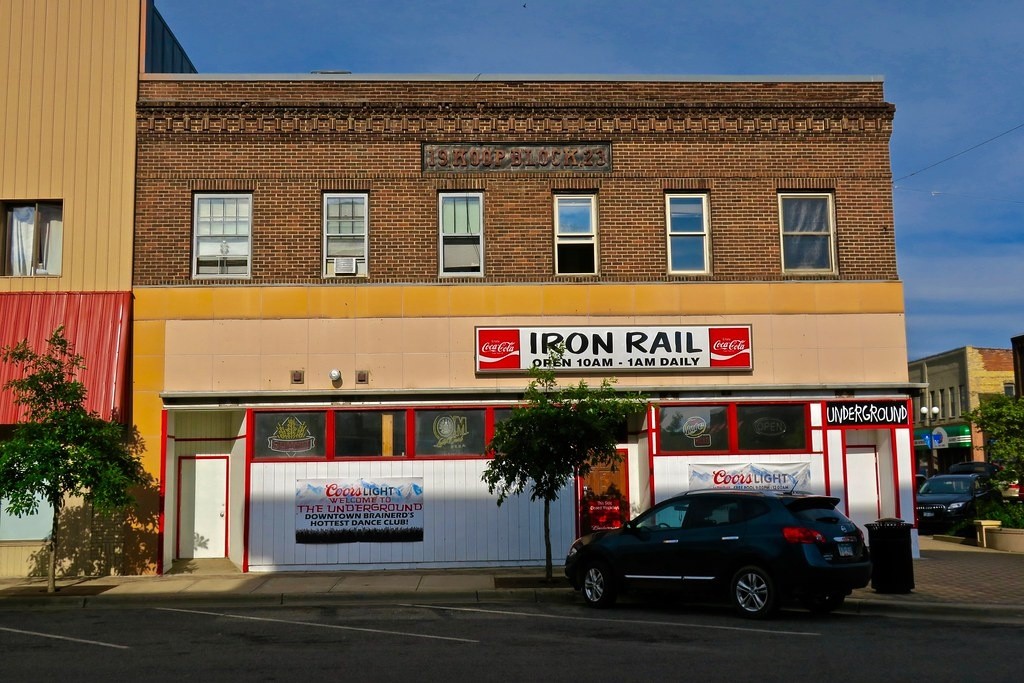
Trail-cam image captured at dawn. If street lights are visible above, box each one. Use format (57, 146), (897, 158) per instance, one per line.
(920, 406), (939, 476)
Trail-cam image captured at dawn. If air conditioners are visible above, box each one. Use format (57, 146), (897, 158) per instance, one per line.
(333, 258), (356, 274)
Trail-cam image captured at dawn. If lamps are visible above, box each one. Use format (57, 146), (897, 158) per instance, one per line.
(330, 369), (341, 380)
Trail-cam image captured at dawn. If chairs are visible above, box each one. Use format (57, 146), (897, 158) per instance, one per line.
(692, 504), (716, 527)
(943, 484), (954, 493)
(729, 507), (755, 523)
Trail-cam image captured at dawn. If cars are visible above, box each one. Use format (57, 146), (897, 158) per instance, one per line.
(915, 474), (1001, 538)
(943, 461), (1005, 484)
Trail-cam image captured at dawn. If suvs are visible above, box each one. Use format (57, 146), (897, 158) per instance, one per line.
(565, 489), (872, 622)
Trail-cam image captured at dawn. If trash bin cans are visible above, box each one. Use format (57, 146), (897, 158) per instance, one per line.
(864, 517), (916, 595)
